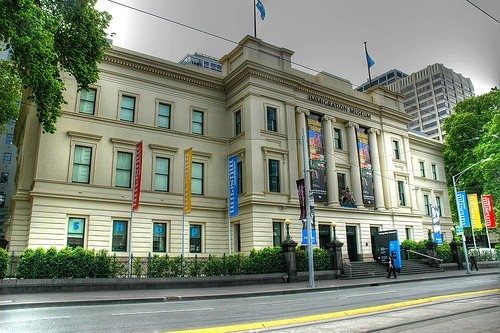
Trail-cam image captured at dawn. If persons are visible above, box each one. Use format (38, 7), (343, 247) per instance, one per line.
(386, 256), (397, 279)
(470, 252), (479, 271)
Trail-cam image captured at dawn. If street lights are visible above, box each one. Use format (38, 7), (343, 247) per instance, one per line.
(282, 218), (298, 276)
(328, 222), (345, 271)
(448, 227), (464, 269)
(452, 157), (494, 274)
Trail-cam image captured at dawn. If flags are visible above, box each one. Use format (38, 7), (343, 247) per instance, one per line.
(228, 154), (238, 216)
(255, 0), (265, 21)
(457, 191), (497, 229)
(296, 178), (305, 221)
(367, 53), (375, 68)
(133, 140), (143, 210)
(184, 146), (191, 214)
